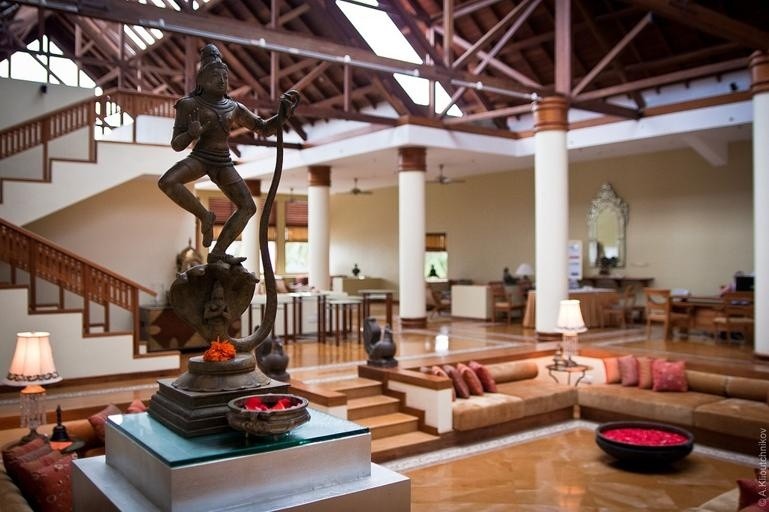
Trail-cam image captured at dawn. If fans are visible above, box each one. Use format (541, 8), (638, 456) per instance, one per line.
(424, 164), (467, 185)
(333, 177), (374, 196)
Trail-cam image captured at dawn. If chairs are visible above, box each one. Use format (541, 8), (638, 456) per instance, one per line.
(425, 278), (754, 348)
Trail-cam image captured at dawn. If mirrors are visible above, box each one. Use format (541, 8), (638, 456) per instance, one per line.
(584, 179), (631, 268)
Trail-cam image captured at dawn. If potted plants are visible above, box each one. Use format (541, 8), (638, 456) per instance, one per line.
(598, 256), (620, 275)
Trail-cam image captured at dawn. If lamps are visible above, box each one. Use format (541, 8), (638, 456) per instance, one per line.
(1, 330), (66, 443)
(553, 296), (592, 364)
(514, 262), (537, 289)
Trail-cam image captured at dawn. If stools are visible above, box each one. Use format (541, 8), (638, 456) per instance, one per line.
(247, 288), (396, 347)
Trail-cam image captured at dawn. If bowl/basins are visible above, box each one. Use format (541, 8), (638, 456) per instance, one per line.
(594, 420), (696, 470)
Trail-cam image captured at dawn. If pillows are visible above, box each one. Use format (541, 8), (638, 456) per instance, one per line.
(419, 359), (497, 401)
(602, 352), (689, 393)
(85, 401), (124, 444)
(2, 435), (79, 512)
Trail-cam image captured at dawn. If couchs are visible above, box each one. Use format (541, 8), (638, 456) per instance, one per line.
(1, 418), (108, 512)
(578, 367), (768, 460)
(453, 362), (578, 447)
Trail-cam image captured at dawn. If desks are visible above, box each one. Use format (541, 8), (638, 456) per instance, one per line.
(544, 361), (595, 387)
(330, 275), (386, 295)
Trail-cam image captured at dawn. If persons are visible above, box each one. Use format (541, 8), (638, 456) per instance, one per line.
(155, 41), (302, 266)
(202, 282), (234, 340)
